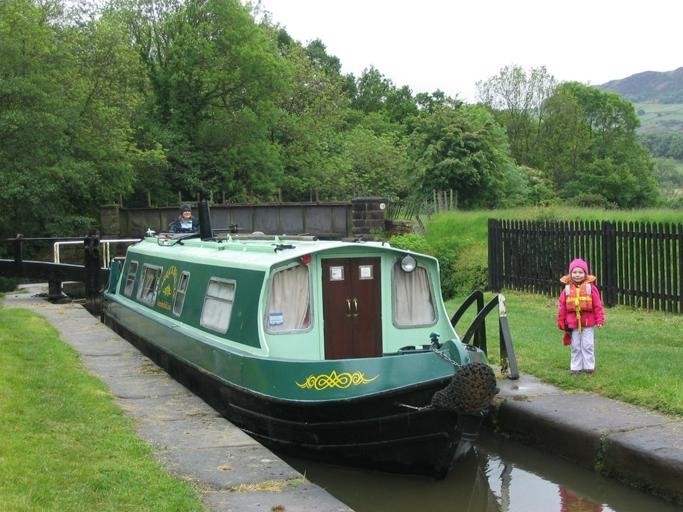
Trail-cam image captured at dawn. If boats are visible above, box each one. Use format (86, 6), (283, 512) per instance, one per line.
(100, 198), (502, 484)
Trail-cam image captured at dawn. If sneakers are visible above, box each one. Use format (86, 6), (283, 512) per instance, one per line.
(571, 369), (593, 373)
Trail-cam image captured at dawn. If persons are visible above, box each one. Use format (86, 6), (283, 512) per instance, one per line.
(168, 204), (200, 233)
(557, 259), (606, 373)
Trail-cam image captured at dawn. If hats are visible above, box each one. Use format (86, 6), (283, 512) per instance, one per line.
(180, 205), (191, 211)
(569, 259), (588, 274)
